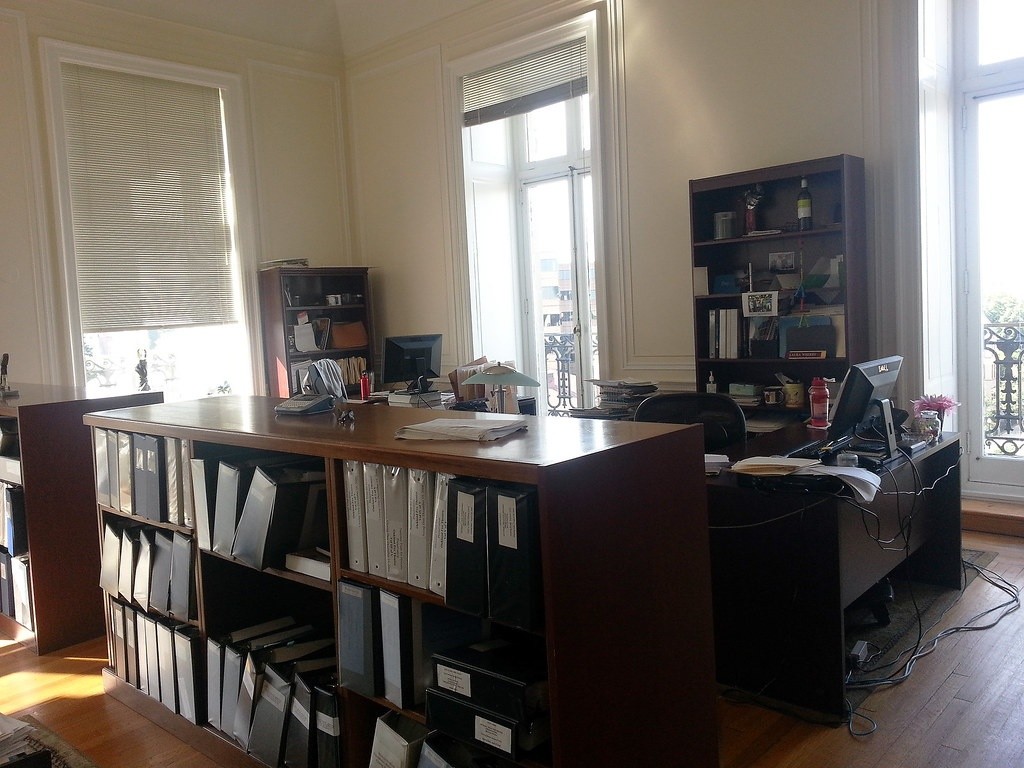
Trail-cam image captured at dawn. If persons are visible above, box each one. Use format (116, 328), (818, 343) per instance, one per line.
(749, 295), (773, 313)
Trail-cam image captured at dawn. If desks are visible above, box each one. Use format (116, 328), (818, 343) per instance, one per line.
(704, 425), (963, 722)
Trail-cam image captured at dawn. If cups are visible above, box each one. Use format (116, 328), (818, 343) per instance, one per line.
(326, 295), (342, 307)
(764, 386), (784, 405)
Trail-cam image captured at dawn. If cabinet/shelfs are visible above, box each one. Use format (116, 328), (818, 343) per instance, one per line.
(82, 395), (721, 768)
(689, 154), (870, 440)
(0, 380), (164, 656)
(257, 267), (376, 398)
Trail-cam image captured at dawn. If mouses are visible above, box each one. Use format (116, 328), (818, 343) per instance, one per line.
(767, 451), (787, 458)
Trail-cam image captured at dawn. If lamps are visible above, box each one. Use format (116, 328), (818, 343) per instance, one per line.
(461, 362), (542, 414)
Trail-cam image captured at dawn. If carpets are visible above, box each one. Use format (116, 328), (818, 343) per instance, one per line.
(716, 546), (999, 730)
(0, 714), (101, 768)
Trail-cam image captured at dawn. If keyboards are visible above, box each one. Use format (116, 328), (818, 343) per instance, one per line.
(787, 435), (853, 460)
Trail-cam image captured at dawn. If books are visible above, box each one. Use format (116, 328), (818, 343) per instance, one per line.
(569, 381), (661, 420)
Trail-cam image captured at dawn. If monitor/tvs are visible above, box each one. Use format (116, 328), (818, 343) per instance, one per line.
(383, 334), (442, 395)
(827, 355), (904, 466)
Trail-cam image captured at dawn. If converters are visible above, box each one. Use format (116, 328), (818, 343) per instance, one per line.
(849, 640), (867, 666)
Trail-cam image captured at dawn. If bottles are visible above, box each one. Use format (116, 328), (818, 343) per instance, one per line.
(918, 410), (941, 442)
(293, 296), (301, 307)
(807, 377), (830, 428)
(783, 383), (805, 408)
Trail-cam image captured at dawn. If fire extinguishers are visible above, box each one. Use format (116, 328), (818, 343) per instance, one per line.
(360, 373), (370, 398)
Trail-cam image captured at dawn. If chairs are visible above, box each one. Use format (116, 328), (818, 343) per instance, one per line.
(633, 393), (747, 454)
